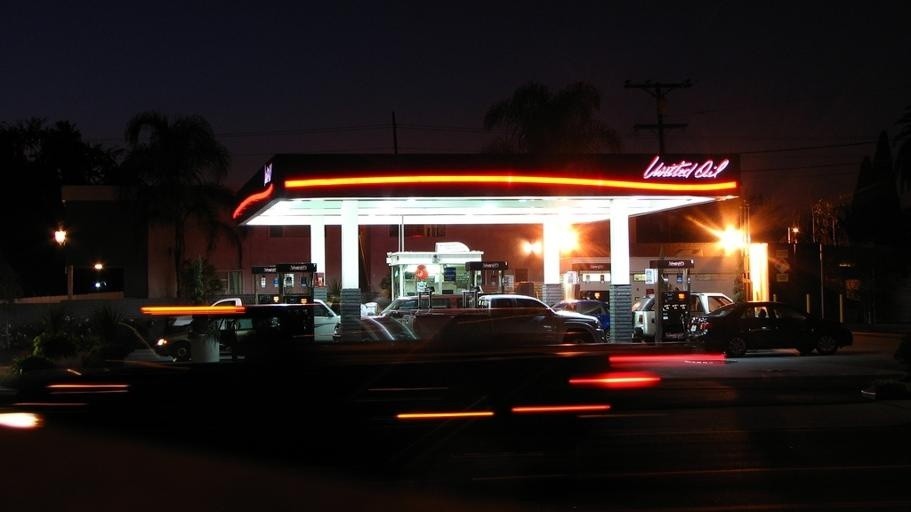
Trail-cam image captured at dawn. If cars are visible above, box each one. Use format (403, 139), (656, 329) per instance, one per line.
(146, 288), (610, 368)
(689, 301), (856, 357)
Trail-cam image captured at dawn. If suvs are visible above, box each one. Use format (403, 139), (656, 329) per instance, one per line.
(633, 292), (736, 339)
